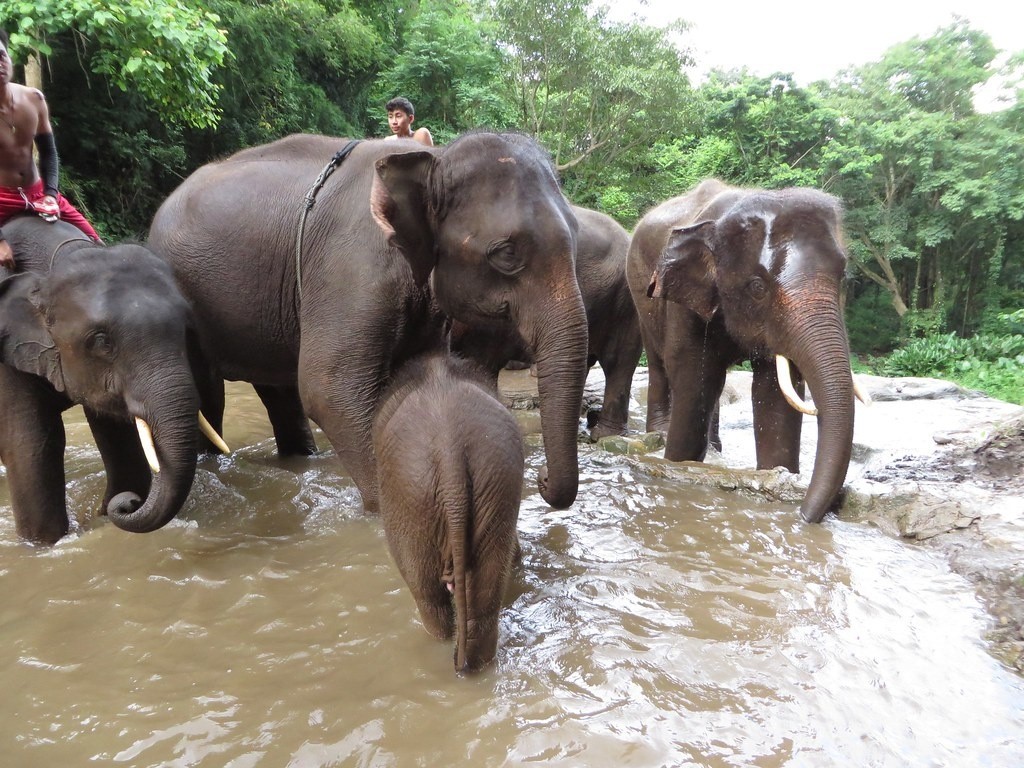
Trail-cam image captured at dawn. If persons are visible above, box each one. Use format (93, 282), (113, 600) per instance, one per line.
(383, 97), (434, 148)
(0, 27), (109, 271)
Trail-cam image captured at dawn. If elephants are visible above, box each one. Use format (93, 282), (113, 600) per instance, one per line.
(0, 133), (867, 676)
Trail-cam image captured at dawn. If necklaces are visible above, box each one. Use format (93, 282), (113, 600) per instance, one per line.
(1, 91), (16, 133)
